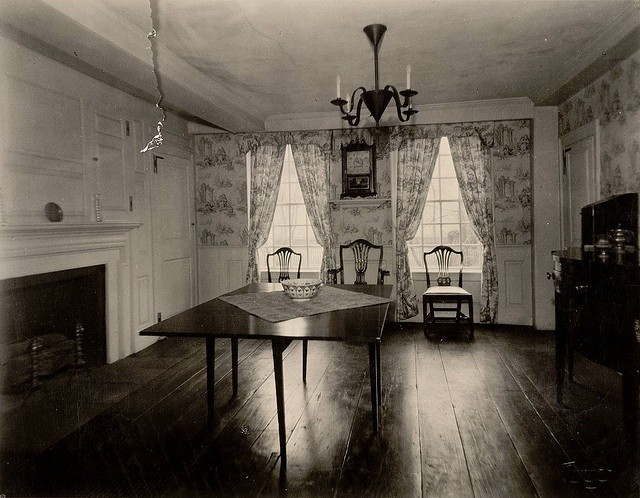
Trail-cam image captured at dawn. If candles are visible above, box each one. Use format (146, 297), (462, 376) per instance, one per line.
(405, 64), (411, 88)
(346, 92), (351, 115)
(335, 75), (342, 100)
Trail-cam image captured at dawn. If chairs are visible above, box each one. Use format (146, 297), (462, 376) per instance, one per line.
(329, 239), (390, 284)
(422, 246), (474, 338)
(266, 247), (302, 282)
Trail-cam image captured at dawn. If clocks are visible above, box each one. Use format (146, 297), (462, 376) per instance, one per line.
(344, 146), (373, 177)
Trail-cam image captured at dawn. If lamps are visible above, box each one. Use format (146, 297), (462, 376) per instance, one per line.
(329, 23), (417, 128)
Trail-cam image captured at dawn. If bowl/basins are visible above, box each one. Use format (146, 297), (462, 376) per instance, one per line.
(280, 277), (321, 303)
